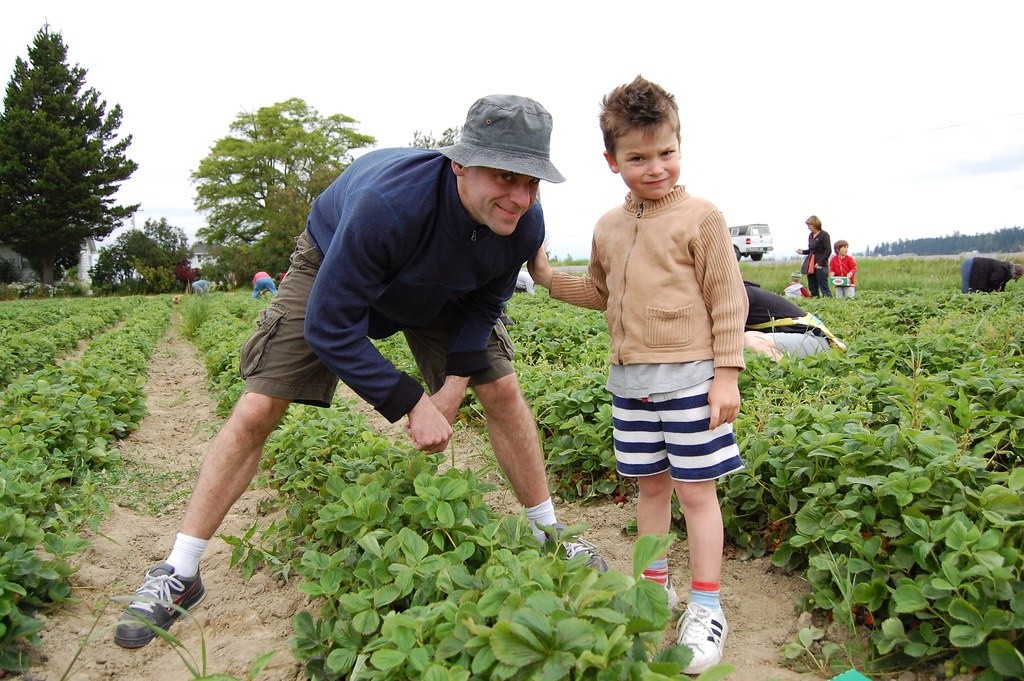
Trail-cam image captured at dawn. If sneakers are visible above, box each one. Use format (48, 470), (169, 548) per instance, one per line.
(676, 602), (729, 674)
(638, 573), (680, 609)
(541, 522), (608, 574)
(114, 563), (207, 648)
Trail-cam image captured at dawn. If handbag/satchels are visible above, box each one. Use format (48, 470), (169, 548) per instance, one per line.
(805, 311), (848, 354)
(801, 251), (814, 275)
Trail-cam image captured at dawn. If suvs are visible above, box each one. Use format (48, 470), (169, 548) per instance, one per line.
(728, 224), (774, 262)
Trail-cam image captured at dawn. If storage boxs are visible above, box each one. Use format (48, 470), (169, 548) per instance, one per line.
(829, 276), (851, 287)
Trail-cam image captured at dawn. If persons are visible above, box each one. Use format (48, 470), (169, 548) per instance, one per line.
(115, 93), (608, 651)
(783, 272), (811, 299)
(795, 215), (833, 298)
(961, 256), (1024, 294)
(527, 75), (748, 674)
(829, 240), (857, 299)
(743, 281), (848, 363)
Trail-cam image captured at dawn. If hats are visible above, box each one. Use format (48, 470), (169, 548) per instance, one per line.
(1014, 264), (1024, 283)
(791, 272), (803, 280)
(438, 94), (567, 184)
